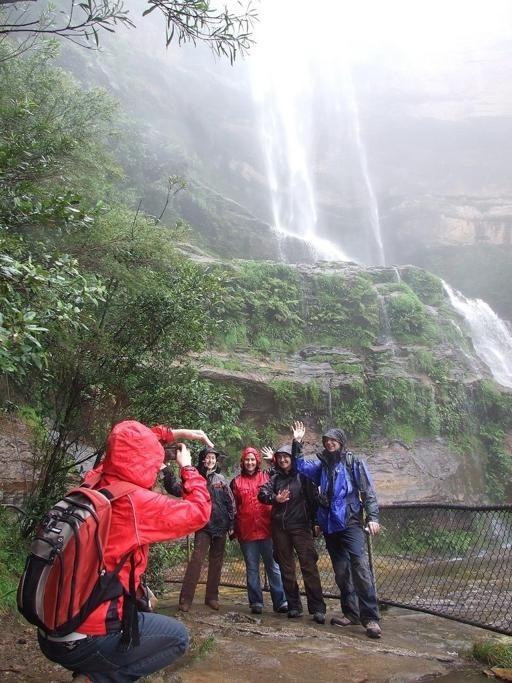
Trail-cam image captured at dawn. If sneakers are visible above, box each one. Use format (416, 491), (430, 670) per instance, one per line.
(366, 622), (382, 639)
(330, 615), (355, 629)
(313, 610), (327, 625)
(250, 605), (263, 614)
(70, 673), (91, 683)
(288, 609), (305, 619)
(204, 597), (219, 610)
(273, 604), (288, 613)
(179, 603), (190, 613)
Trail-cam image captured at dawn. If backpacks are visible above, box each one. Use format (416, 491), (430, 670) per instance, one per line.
(16, 473), (136, 638)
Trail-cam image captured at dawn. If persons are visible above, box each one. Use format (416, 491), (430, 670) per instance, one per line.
(256, 443), (327, 625)
(37, 417), (215, 683)
(290, 418), (384, 640)
(228, 447), (287, 616)
(157, 447), (237, 613)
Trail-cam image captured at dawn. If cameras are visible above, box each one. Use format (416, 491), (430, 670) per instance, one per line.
(163, 447), (182, 460)
